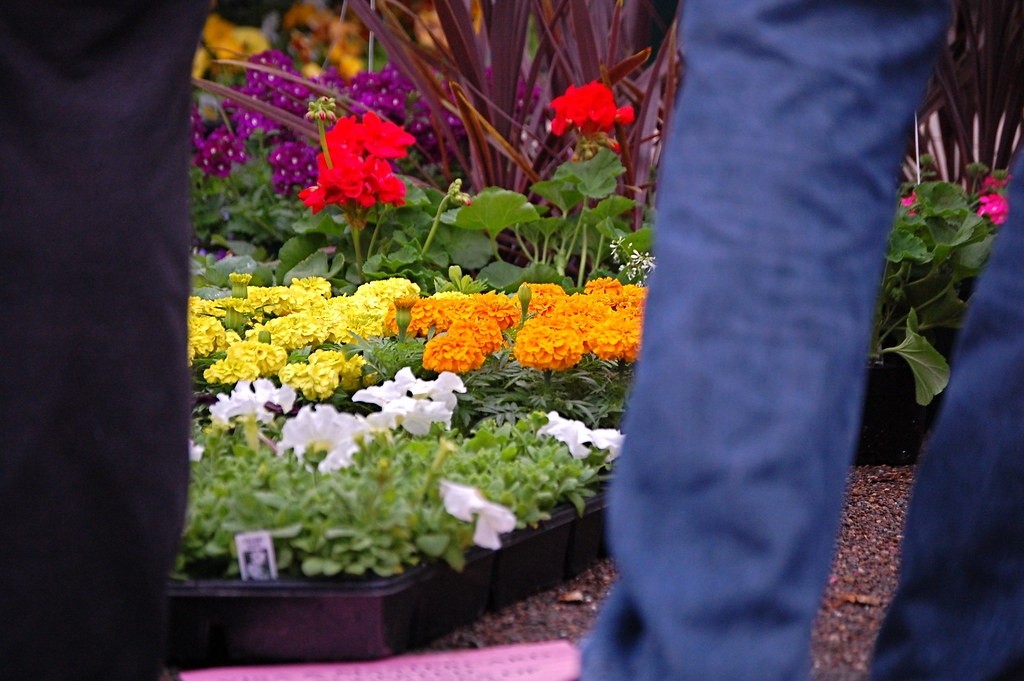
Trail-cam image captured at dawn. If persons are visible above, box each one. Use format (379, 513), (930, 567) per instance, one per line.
(577, 0), (1024, 681)
(0, 0), (214, 681)
(245, 538), (269, 580)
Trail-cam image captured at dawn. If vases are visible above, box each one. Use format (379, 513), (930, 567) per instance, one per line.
(161, 490), (608, 667)
(855, 340), (932, 464)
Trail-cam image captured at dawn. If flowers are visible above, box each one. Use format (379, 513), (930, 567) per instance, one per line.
(862, 151), (1014, 403)
(169, 0), (660, 580)
